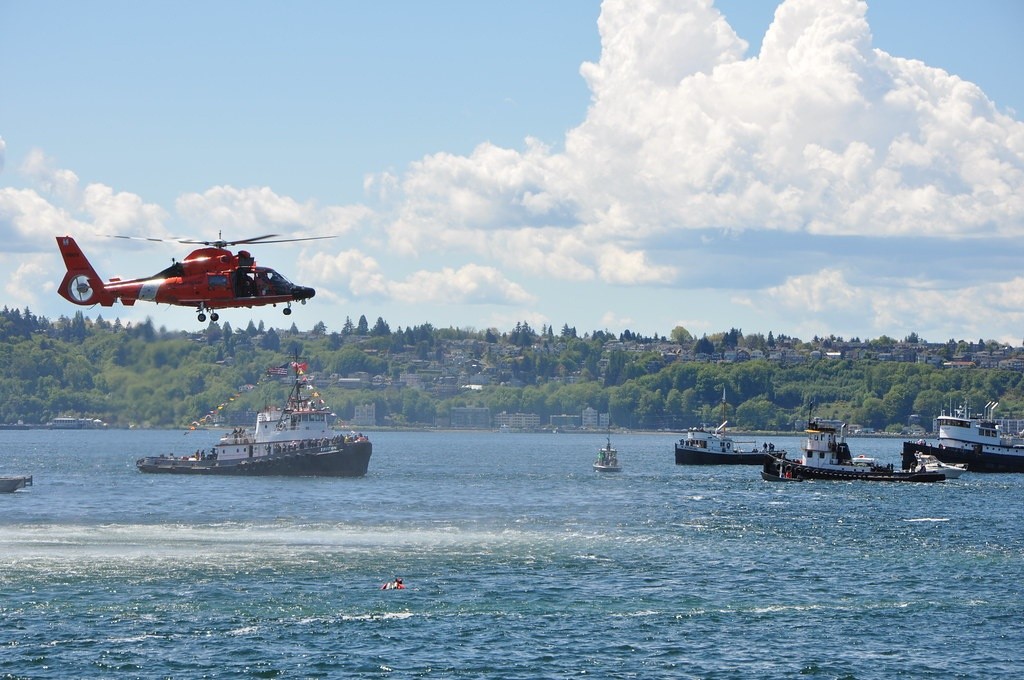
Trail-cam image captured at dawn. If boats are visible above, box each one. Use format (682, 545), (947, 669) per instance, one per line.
(759, 402), (944, 482)
(675, 388), (786, 466)
(0, 474), (34, 494)
(893, 454), (969, 478)
(937, 415), (1024, 473)
(593, 430), (622, 473)
(759, 470), (803, 484)
(136, 348), (374, 477)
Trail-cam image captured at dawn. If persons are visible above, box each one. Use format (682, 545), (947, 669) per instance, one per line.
(232, 426), (245, 435)
(596, 451), (610, 466)
(264, 430), (369, 455)
(195, 449), (206, 460)
(917, 438), (932, 447)
(679, 438), (693, 448)
(918, 463), (925, 473)
(763, 442), (775, 453)
(909, 461), (916, 472)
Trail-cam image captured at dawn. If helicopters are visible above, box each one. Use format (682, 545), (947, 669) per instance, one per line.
(55, 231), (341, 321)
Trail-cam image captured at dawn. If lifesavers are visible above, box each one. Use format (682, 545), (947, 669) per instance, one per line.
(243, 452), (311, 471)
(776, 464), (867, 480)
(727, 443), (730, 449)
(279, 423), (284, 430)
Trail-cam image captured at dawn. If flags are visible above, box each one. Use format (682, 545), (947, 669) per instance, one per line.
(268, 361), (289, 375)
